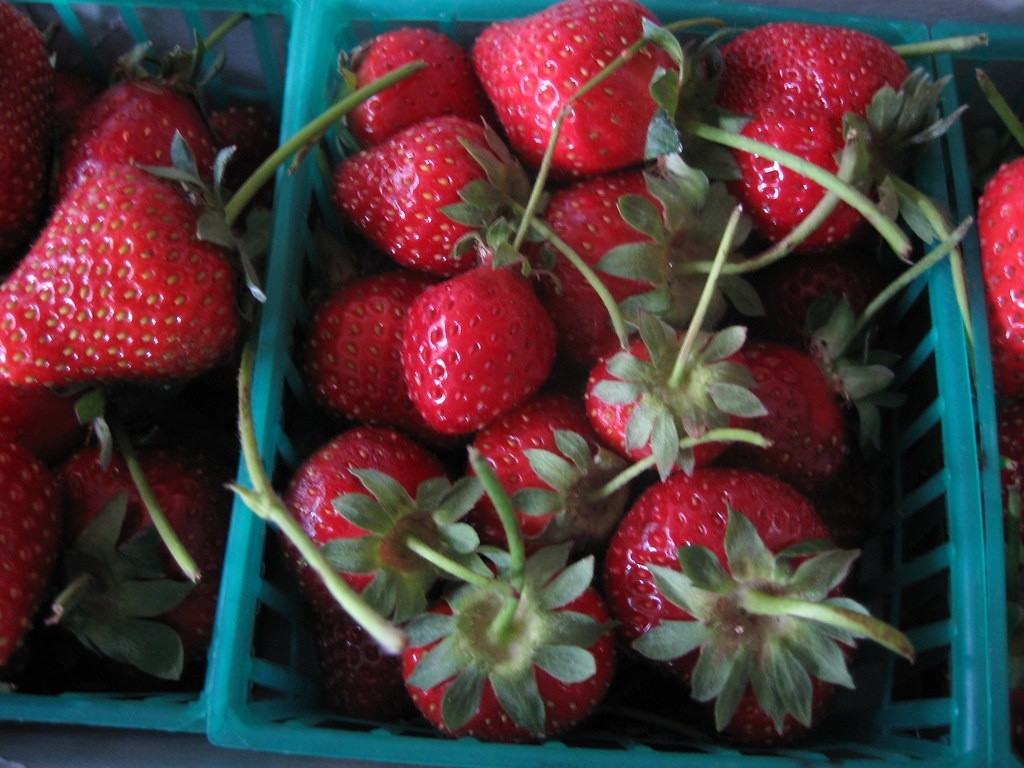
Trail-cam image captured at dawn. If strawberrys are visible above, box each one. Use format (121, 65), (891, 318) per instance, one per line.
(0, 1), (1024, 738)
